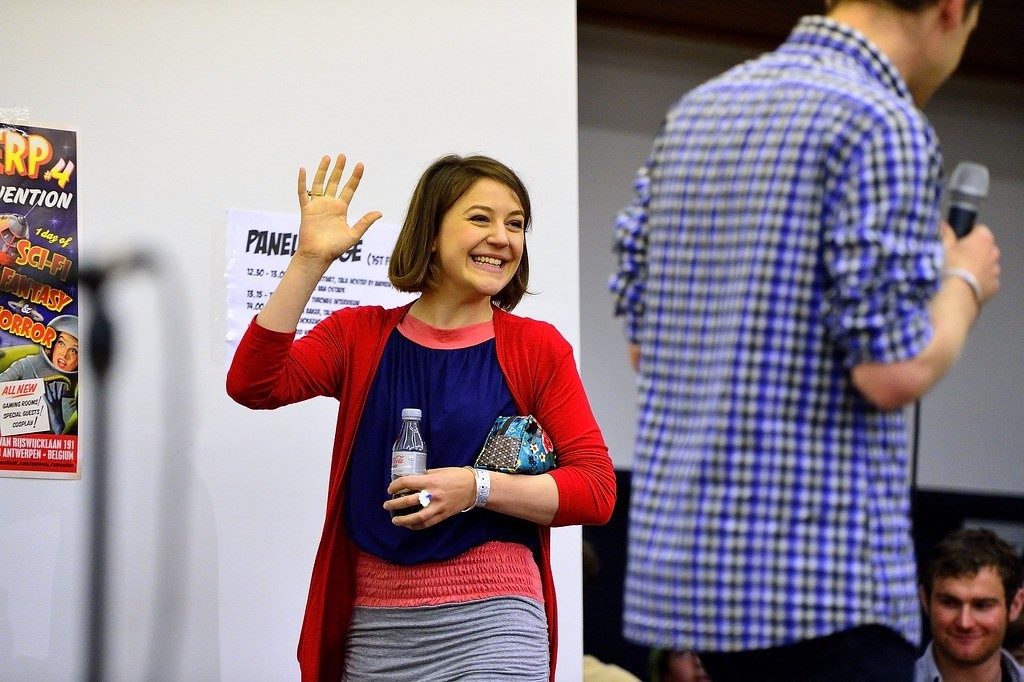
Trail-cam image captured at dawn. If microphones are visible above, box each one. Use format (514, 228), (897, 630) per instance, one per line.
(945, 162), (990, 240)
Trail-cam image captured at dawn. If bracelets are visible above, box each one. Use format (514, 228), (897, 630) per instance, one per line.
(461, 465), (490, 513)
(943, 267), (984, 316)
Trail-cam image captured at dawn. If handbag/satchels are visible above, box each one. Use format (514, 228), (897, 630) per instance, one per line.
(473, 415), (559, 475)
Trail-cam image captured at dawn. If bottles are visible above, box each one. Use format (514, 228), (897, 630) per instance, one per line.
(391, 408), (427, 522)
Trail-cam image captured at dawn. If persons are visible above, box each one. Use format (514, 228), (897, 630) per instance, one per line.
(650, 644), (709, 681)
(226, 153), (618, 682)
(608, 0), (1024, 682)
(914, 527), (1024, 682)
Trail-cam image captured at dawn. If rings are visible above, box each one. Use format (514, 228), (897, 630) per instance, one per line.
(307, 190), (323, 196)
(418, 489), (432, 508)
